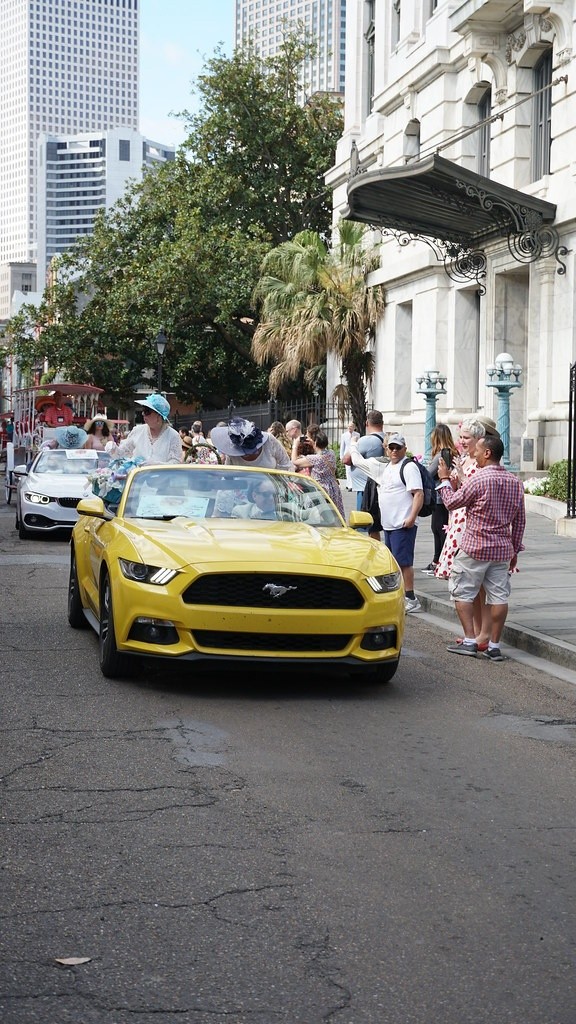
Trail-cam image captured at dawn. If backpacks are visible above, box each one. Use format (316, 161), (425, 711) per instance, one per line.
(399, 457), (437, 517)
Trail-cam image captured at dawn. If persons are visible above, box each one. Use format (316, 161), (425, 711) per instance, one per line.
(232, 477), (281, 521)
(438, 417), (525, 661)
(212, 413), (295, 521)
(105, 393), (189, 514)
(0, 390), (423, 616)
(421, 423), (460, 578)
(85, 410), (113, 450)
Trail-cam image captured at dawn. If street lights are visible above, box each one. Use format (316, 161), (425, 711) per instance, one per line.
(415, 368), (448, 472)
(155, 323), (168, 395)
(486, 352), (524, 474)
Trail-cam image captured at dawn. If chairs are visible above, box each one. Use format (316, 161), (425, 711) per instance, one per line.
(210, 477), (256, 519)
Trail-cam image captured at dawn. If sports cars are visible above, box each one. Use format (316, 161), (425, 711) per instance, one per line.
(66, 464), (407, 687)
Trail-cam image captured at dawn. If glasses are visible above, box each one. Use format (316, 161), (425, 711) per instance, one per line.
(94, 421), (105, 427)
(255, 491), (278, 499)
(286, 427), (298, 434)
(387, 444), (403, 451)
(142, 407), (156, 416)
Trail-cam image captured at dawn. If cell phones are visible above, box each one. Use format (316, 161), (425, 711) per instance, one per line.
(300, 437), (308, 442)
(442, 448), (450, 470)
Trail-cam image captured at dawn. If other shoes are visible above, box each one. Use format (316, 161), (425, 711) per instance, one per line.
(346, 487), (352, 491)
(477, 641), (489, 651)
(457, 639), (462, 643)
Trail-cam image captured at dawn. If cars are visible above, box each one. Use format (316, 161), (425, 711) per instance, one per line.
(10, 447), (117, 539)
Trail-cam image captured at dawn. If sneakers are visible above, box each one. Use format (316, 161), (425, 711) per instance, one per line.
(405, 596), (422, 614)
(483, 646), (502, 660)
(446, 641), (478, 656)
(420, 563), (437, 576)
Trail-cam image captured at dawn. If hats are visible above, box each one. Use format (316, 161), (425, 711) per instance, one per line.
(134, 395), (171, 421)
(56, 425), (89, 449)
(85, 413), (113, 432)
(210, 416), (269, 456)
(388, 435), (406, 447)
(53, 390), (62, 397)
(181, 436), (192, 448)
(476, 415), (501, 438)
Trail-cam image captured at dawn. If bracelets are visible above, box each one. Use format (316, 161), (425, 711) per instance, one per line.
(439, 477), (451, 482)
